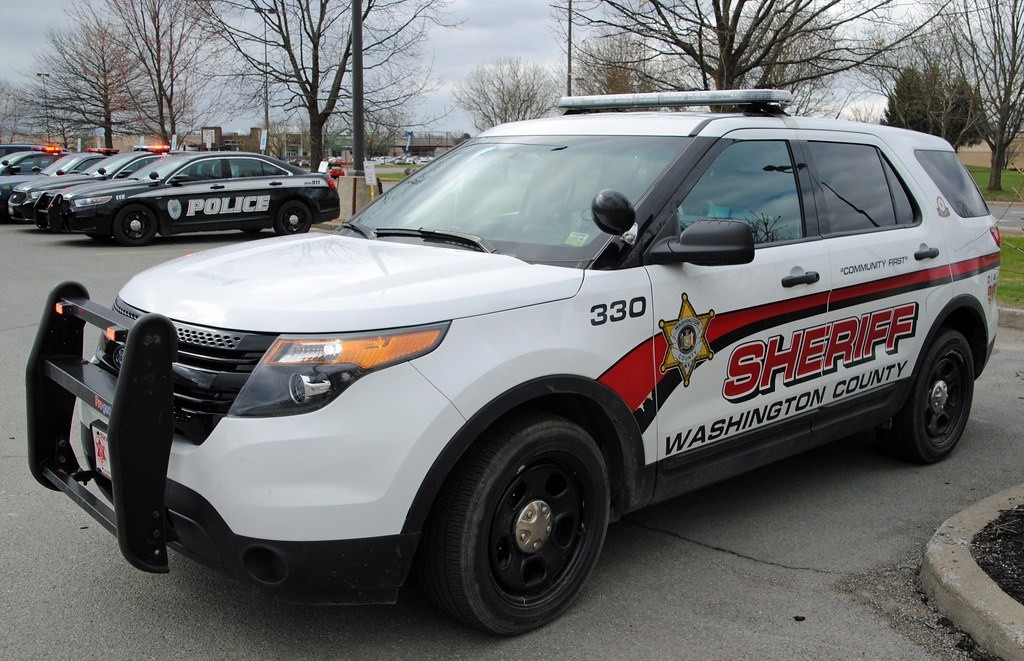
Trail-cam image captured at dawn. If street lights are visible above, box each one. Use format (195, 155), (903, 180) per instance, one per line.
(36, 73), (51, 145)
(253, 6), (278, 155)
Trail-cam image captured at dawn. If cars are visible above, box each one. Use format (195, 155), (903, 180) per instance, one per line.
(0, 147), (121, 219)
(0, 145), (72, 177)
(0, 144), (50, 171)
(8, 144), (239, 226)
(32, 151), (340, 247)
(287, 153), (434, 169)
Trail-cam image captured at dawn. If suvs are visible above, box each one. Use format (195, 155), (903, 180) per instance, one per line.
(22, 87), (1002, 637)
